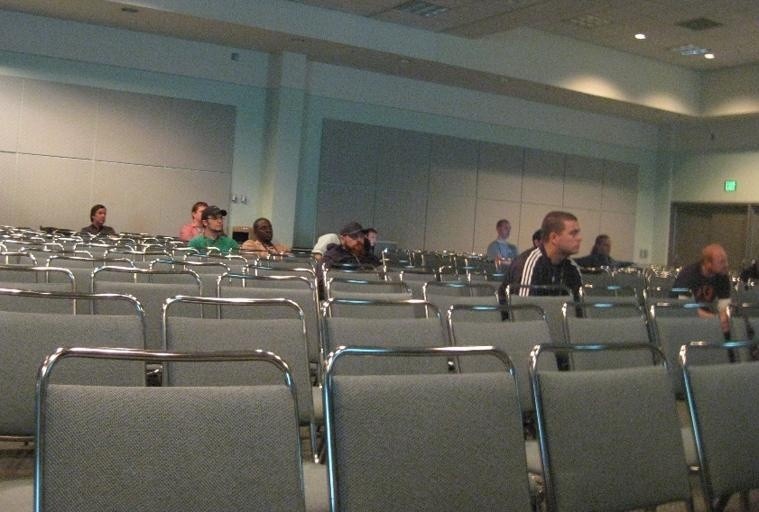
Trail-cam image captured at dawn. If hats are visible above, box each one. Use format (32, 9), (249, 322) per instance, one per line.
(201, 206), (227, 220)
(340, 223), (369, 236)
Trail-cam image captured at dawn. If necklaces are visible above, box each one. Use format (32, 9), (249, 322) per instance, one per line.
(261, 241), (279, 256)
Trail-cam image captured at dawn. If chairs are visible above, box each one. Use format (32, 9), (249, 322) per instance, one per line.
(2, 227), (758, 510)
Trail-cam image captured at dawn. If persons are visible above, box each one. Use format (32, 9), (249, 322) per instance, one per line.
(740, 259), (759, 288)
(188, 206), (239, 255)
(181, 203), (208, 241)
(310, 221), (379, 299)
(80, 205), (115, 237)
(589, 235), (616, 266)
(487, 220), (517, 262)
(498, 211), (585, 321)
(669, 244), (731, 334)
(241, 218), (288, 259)
(533, 230), (542, 248)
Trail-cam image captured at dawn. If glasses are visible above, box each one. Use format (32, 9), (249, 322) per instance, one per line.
(208, 216), (223, 221)
